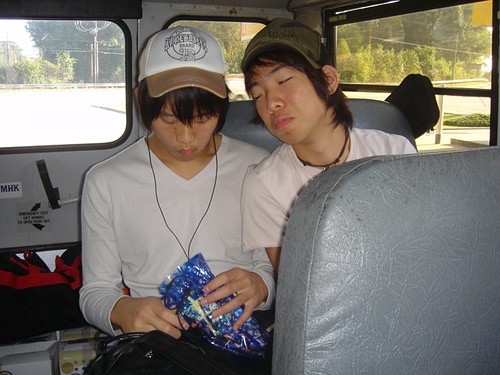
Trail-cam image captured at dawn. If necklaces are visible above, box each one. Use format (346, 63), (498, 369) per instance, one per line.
(296, 122), (349, 171)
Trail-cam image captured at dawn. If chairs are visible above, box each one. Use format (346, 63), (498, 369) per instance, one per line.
(213, 98), (423, 167)
(268, 145), (500, 375)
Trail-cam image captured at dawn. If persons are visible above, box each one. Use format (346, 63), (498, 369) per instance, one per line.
(241, 20), (416, 270)
(78, 26), (273, 375)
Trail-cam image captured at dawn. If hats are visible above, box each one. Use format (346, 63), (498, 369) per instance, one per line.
(240, 17), (334, 70)
(138, 24), (227, 99)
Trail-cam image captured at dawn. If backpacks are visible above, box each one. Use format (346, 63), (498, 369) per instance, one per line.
(82, 329), (272, 374)
